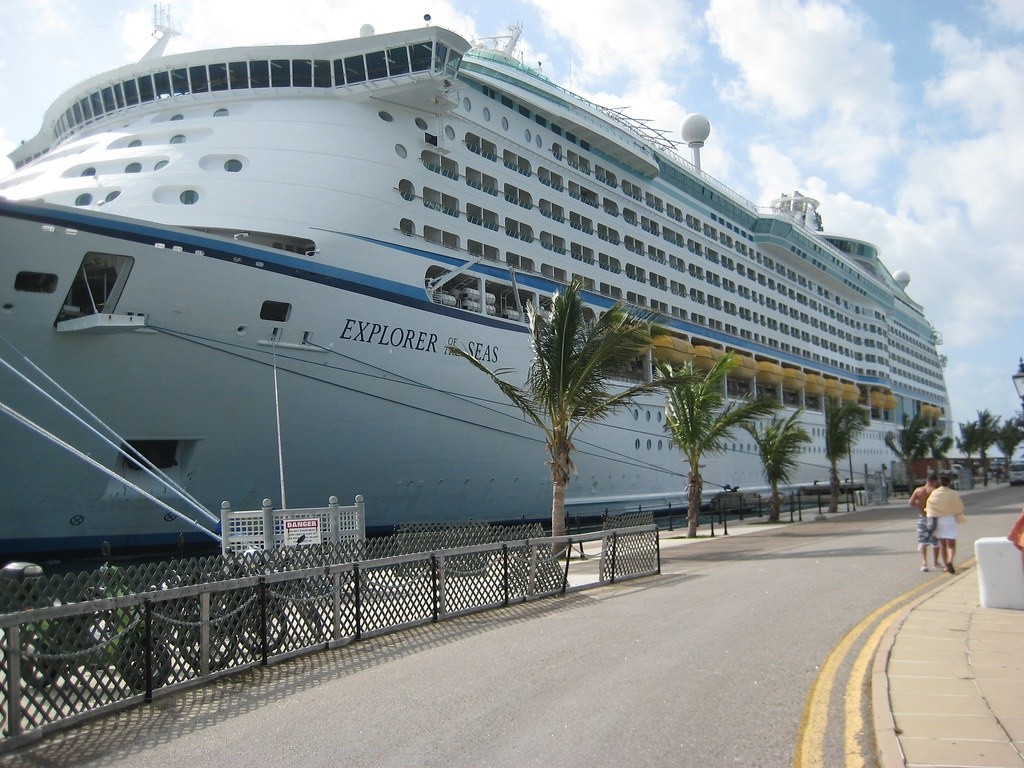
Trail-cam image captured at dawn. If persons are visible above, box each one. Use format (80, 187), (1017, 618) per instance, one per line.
(909, 474), (964, 574)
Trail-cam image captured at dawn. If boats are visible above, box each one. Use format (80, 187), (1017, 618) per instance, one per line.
(781, 366), (808, 392)
(805, 373), (828, 395)
(693, 344), (727, 372)
(756, 361), (785, 386)
(842, 383), (861, 401)
(923, 405), (940, 420)
(726, 351), (760, 380)
(650, 334), (695, 365)
(870, 390), (886, 408)
(825, 378), (845, 399)
(884, 392), (897, 411)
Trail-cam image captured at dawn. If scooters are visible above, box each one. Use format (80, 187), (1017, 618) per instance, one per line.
(0, 532), (325, 694)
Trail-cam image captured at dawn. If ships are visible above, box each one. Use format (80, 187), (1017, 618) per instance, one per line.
(0, 0), (957, 555)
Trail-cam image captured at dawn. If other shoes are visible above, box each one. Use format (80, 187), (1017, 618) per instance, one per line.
(933, 564), (942, 568)
(946, 562), (955, 574)
(922, 566), (928, 572)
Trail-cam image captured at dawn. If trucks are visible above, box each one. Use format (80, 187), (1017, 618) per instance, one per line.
(1008, 462), (1024, 485)
